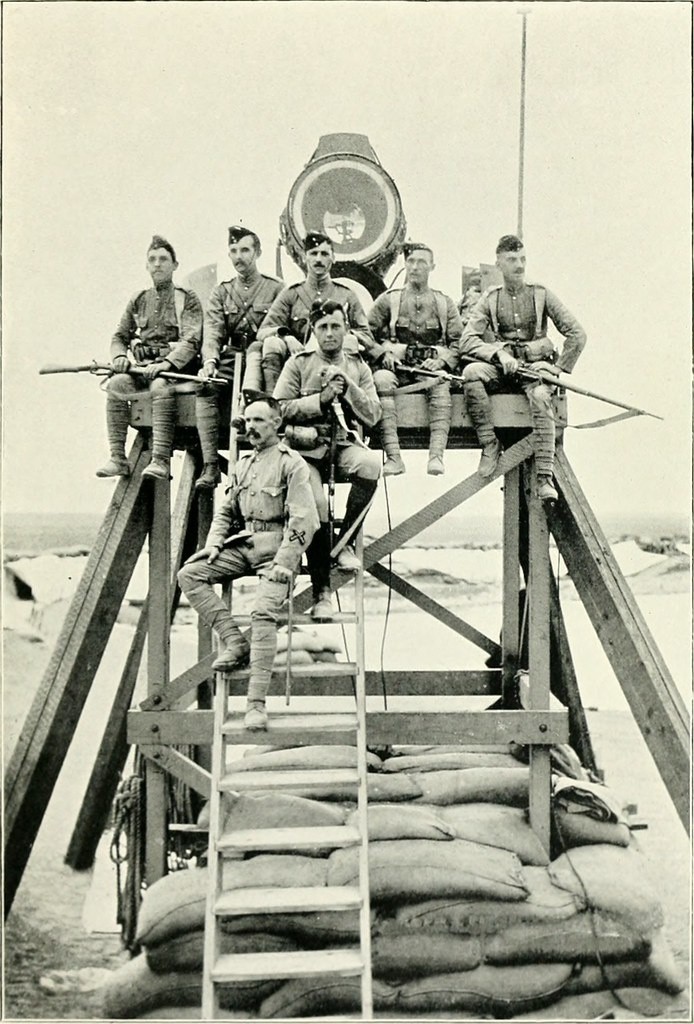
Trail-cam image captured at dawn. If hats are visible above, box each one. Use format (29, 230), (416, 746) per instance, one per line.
(228, 226), (261, 247)
(304, 231), (332, 249)
(496, 234), (524, 254)
(403, 242), (433, 258)
(147, 234), (176, 264)
(309, 297), (347, 325)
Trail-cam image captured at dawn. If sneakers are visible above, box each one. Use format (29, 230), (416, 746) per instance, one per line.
(243, 701), (267, 729)
(478, 438), (502, 476)
(194, 465), (221, 489)
(382, 454), (405, 476)
(535, 474), (558, 502)
(142, 457), (170, 480)
(96, 457), (130, 478)
(338, 547), (362, 572)
(427, 454), (444, 475)
(310, 592), (333, 619)
(230, 415), (246, 433)
(212, 641), (250, 672)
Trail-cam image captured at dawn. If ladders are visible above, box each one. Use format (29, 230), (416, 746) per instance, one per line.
(201, 352), (373, 1021)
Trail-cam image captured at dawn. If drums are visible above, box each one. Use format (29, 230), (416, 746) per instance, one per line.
(280, 152), (407, 280)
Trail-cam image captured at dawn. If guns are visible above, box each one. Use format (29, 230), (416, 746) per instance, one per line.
(39, 364), (229, 384)
(461, 354), (664, 421)
(359, 350), (466, 381)
(328, 394), (338, 569)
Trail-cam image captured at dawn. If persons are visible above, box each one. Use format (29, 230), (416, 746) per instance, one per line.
(258, 232), (374, 396)
(97, 236), (203, 479)
(177, 396), (320, 730)
(194, 226), (286, 489)
(368, 243), (464, 477)
(458, 235), (587, 501)
(273, 301), (382, 621)
